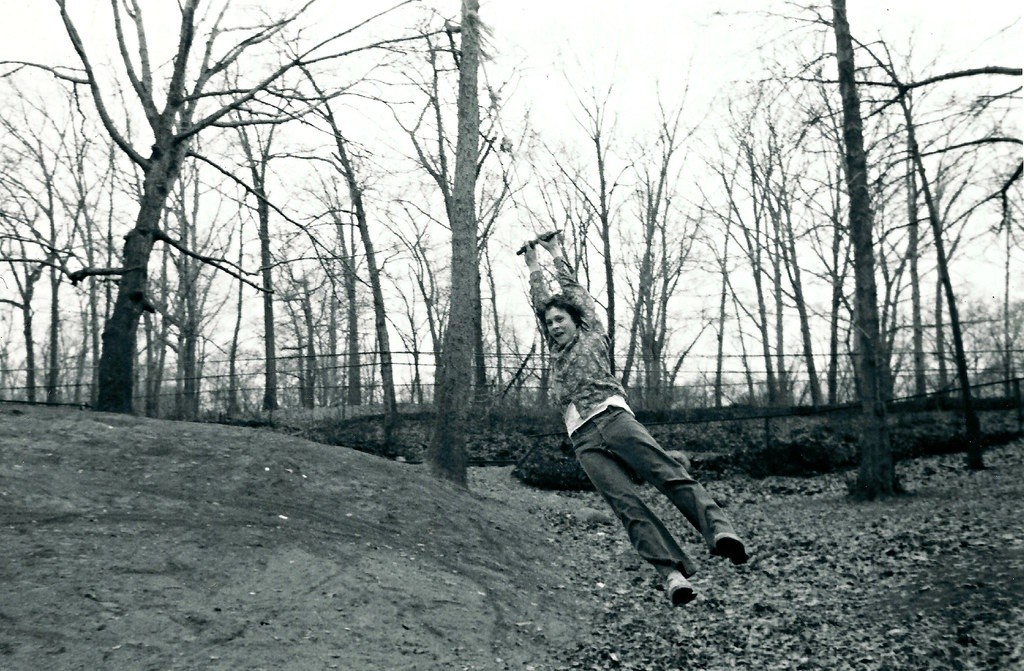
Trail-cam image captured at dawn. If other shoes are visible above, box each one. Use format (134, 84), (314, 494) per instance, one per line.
(666, 572), (698, 606)
(715, 532), (749, 565)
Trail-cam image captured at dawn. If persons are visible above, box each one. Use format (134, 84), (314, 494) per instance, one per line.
(521, 231), (751, 607)
